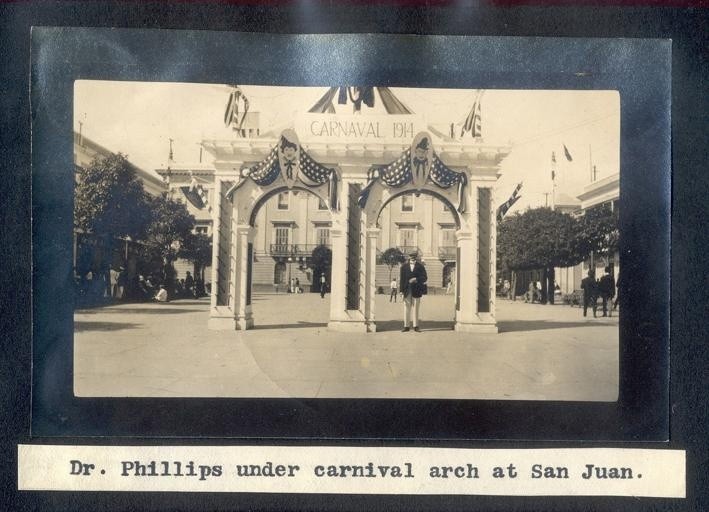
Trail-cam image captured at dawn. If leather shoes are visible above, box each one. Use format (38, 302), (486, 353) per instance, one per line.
(402, 326), (421, 332)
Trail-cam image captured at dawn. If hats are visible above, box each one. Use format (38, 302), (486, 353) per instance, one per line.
(409, 250), (418, 255)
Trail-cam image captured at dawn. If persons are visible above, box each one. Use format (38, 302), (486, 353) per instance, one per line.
(597, 267), (615, 317)
(319, 272), (328, 299)
(448, 282), (454, 294)
(446, 279), (450, 294)
(389, 277), (397, 303)
(290, 278), (295, 293)
(400, 251), (428, 334)
(610, 272), (620, 310)
(294, 278), (300, 293)
(73, 260), (206, 307)
(580, 270), (598, 318)
(288, 277), (290, 292)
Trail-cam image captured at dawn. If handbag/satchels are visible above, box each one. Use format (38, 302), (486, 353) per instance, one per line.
(415, 283), (428, 296)
